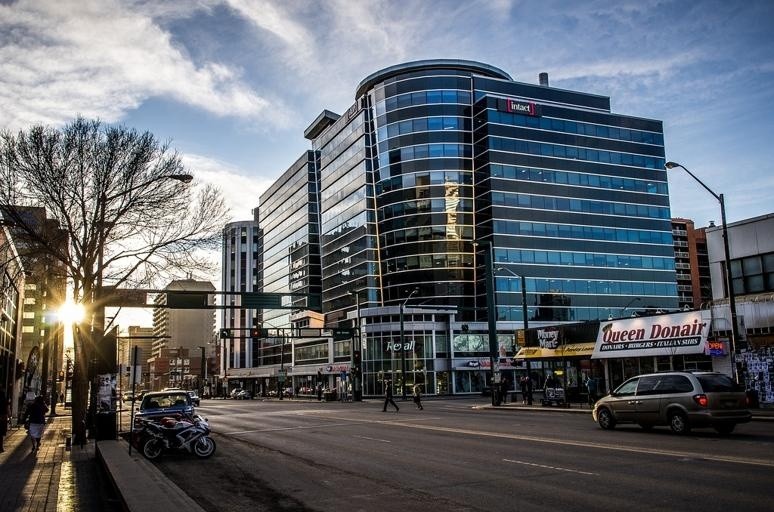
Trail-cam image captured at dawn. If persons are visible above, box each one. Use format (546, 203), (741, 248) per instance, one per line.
(526, 376), (533, 405)
(585, 374), (595, 404)
(295, 385), (300, 398)
(519, 375), (526, 400)
(55, 392), (58, 403)
(501, 380), (508, 402)
(60, 393), (64, 403)
(317, 385), (322, 401)
(0, 391), (8, 453)
(382, 382), (399, 412)
(25, 395), (49, 449)
(543, 376), (553, 394)
(412, 382), (423, 411)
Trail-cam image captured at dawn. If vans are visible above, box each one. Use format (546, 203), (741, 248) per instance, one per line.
(592, 371), (752, 434)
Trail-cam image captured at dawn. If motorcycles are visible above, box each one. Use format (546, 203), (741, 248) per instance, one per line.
(134, 411), (216, 460)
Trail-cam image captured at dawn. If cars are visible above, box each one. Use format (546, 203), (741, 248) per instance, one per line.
(116, 386), (200, 407)
(135, 390), (195, 442)
(258, 387), (328, 396)
(238, 390), (251, 399)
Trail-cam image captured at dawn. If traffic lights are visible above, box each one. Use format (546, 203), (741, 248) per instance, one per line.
(59, 370), (64, 381)
(251, 329), (259, 336)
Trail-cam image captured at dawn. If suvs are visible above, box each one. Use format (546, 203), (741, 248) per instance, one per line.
(230, 388), (243, 399)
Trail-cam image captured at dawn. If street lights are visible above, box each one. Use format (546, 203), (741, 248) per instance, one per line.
(208, 340), (228, 400)
(399, 286), (421, 402)
(347, 287), (364, 401)
(664, 160), (747, 387)
(494, 266), (533, 406)
(257, 320), (285, 401)
(89, 173), (195, 438)
(619, 296), (642, 318)
(196, 346), (205, 380)
